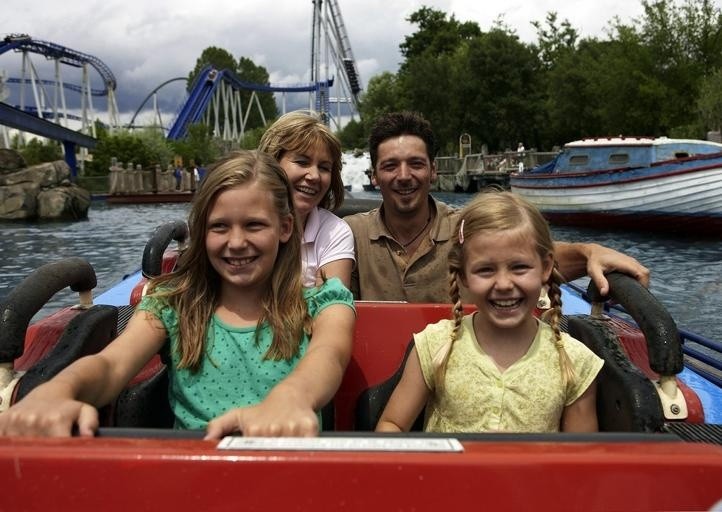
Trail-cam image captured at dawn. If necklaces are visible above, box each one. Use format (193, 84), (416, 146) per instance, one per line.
(376, 204), (432, 246)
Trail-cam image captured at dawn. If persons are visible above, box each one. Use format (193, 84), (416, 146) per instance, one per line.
(165, 163), (183, 191)
(0, 150), (357, 440)
(258, 108), (356, 302)
(343, 110), (650, 304)
(374, 184), (605, 433)
(189, 162), (207, 192)
(512, 142), (527, 164)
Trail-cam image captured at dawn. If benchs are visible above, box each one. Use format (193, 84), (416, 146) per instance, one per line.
(10, 304), (664, 440)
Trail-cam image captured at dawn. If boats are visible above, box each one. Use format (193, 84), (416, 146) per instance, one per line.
(501, 133), (721, 229)
(0, 200), (722, 511)
